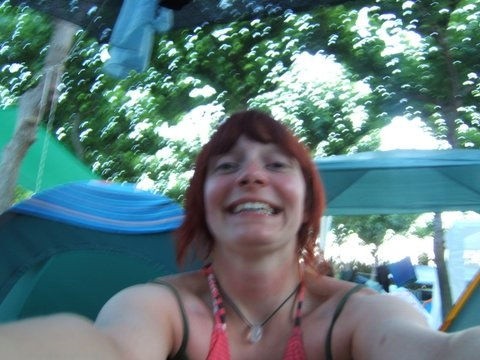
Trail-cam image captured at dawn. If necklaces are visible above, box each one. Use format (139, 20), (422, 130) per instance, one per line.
(216, 278), (302, 344)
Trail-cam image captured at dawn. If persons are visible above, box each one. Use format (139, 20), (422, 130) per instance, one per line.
(366, 265), (429, 320)
(0, 108), (480, 359)
